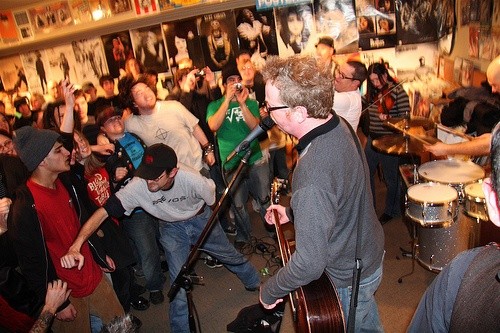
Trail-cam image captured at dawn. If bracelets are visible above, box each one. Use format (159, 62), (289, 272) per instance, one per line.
(202, 142), (214, 157)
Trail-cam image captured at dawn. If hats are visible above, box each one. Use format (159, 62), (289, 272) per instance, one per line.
(134, 143), (177, 180)
(221, 61), (244, 83)
(315, 37), (337, 55)
(94, 103), (122, 127)
(13, 126), (60, 172)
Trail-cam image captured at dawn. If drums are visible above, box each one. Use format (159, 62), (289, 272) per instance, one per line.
(410, 158), (487, 274)
(460, 178), (490, 224)
(403, 179), (460, 231)
(435, 125), (476, 161)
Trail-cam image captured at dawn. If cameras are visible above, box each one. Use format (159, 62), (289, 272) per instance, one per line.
(196, 70), (205, 76)
(234, 83), (243, 91)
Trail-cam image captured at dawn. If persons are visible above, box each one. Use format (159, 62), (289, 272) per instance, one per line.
(112, 37), (127, 70)
(235, 7), (274, 60)
(359, 63), (411, 226)
(331, 60), (368, 134)
(168, 32), (196, 71)
(258, 55), (386, 333)
(356, 0), (397, 36)
(141, 29), (163, 73)
(423, 56), (500, 157)
(407, 121), (500, 333)
(277, 5), (314, 59)
(207, 21), (233, 71)
(0, 49), (291, 333)
(314, 38), (339, 78)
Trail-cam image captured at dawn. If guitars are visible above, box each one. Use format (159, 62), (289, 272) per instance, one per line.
(266, 175), (347, 333)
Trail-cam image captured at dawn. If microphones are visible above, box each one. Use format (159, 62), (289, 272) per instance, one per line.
(224, 117), (275, 165)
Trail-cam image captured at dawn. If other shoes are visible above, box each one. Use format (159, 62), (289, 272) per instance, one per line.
(131, 296), (149, 311)
(379, 214), (392, 224)
(223, 226), (238, 236)
(256, 240), (267, 253)
(133, 265), (145, 278)
(147, 274), (167, 290)
(235, 240), (245, 248)
(149, 291), (164, 304)
(161, 261), (169, 271)
(204, 256), (223, 268)
(133, 284), (146, 294)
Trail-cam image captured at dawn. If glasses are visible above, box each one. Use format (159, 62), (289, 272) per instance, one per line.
(334, 68), (361, 81)
(265, 102), (290, 113)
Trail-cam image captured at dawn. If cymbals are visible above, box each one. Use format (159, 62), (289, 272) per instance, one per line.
(372, 133), (441, 153)
(383, 115), (436, 132)
